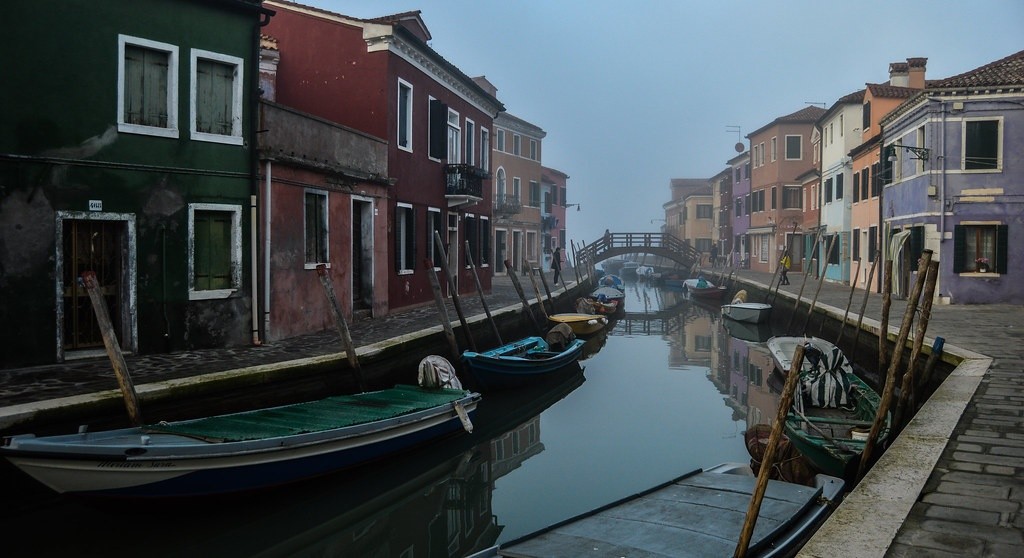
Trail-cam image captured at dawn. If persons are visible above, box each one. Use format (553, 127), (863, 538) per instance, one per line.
(603, 229), (610, 252)
(711, 244), (718, 268)
(551, 247), (561, 286)
(779, 250), (791, 285)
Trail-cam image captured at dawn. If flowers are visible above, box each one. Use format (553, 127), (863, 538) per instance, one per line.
(975, 258), (989, 268)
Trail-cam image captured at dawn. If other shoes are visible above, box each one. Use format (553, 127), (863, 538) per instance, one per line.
(786, 282), (790, 285)
(780, 282), (785, 285)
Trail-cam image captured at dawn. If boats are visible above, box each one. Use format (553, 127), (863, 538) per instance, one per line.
(462, 459), (846, 558)
(574, 297), (619, 317)
(548, 311), (609, 334)
(464, 323), (588, 383)
(779, 342), (892, 479)
(766, 330), (853, 382)
(593, 257), (689, 303)
(683, 275), (727, 299)
(721, 289), (773, 323)
(0, 353), (482, 516)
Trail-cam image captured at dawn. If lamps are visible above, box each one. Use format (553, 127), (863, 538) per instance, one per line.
(887, 145), (930, 161)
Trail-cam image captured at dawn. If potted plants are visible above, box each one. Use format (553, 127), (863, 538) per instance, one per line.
(979, 267), (986, 272)
